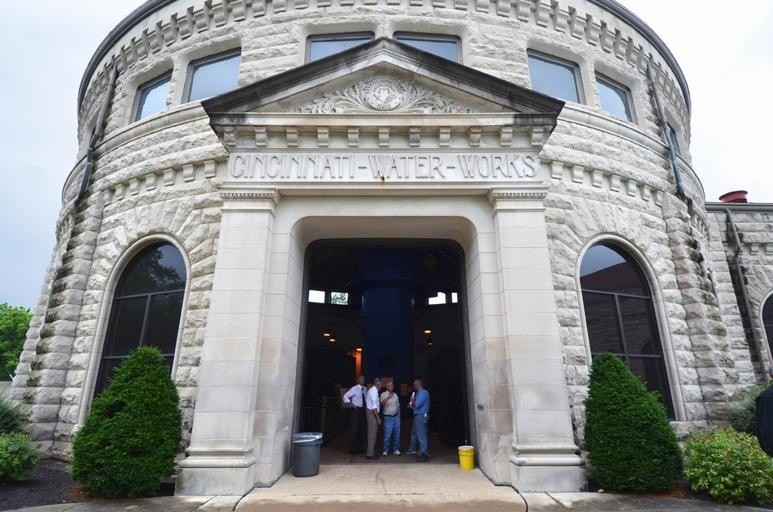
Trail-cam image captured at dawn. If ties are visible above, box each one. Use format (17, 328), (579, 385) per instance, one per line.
(362, 388), (366, 409)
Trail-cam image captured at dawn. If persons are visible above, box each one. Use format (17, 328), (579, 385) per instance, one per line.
(365, 377), (382, 460)
(378, 381), (403, 456)
(406, 379), (430, 457)
(342, 375), (368, 457)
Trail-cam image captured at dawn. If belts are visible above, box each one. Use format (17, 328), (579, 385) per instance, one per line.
(383, 413), (399, 417)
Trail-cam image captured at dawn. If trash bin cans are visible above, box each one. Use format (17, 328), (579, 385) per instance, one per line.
(292, 432), (323, 477)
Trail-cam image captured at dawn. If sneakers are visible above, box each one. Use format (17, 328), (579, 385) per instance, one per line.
(366, 450), (429, 463)
